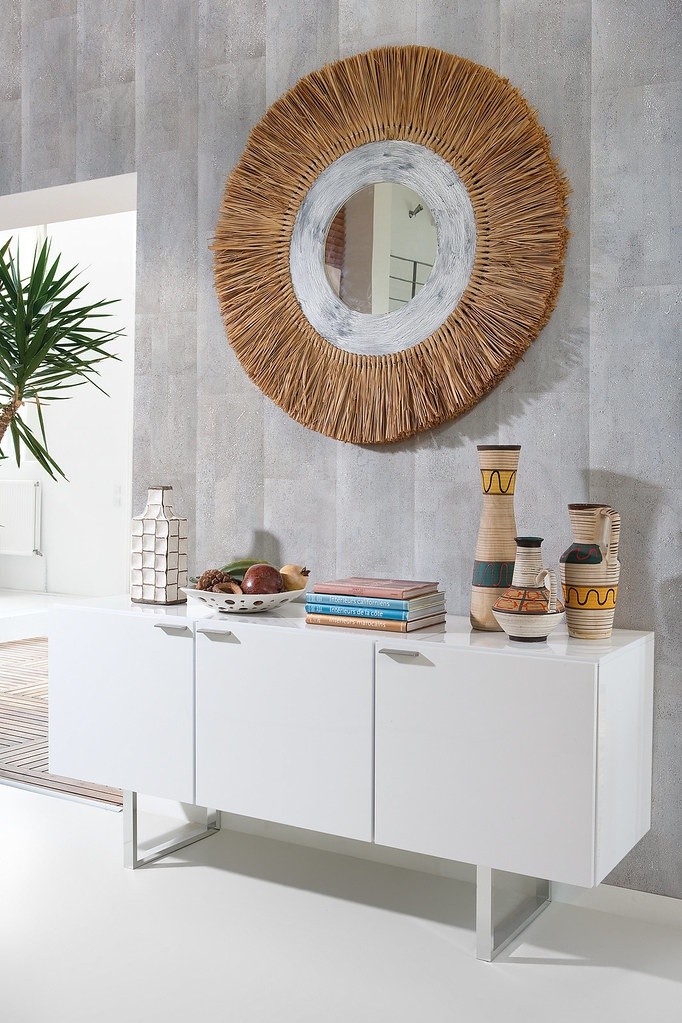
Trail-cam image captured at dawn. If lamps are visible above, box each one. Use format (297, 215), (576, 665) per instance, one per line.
(409, 204), (423, 218)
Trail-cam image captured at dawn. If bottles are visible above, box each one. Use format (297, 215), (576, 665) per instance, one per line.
(130, 482), (187, 606)
(470, 443), (524, 632)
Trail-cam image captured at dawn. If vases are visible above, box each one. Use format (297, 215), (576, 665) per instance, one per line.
(492, 536), (566, 643)
(470, 445), (522, 632)
(558, 502), (621, 639)
(129, 486), (189, 605)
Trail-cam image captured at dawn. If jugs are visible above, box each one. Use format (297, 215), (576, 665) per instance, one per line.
(493, 537), (564, 647)
(560, 503), (622, 641)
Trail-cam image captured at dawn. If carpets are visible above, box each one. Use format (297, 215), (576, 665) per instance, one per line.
(0, 636), (124, 813)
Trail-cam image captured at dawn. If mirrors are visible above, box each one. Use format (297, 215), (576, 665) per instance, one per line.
(287, 141), (476, 356)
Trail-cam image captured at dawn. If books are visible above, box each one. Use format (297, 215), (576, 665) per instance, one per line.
(305, 577), (447, 633)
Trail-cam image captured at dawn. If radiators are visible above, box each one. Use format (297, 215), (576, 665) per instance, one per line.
(0, 479), (43, 557)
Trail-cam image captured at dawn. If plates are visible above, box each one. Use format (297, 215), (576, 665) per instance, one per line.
(175, 576), (305, 614)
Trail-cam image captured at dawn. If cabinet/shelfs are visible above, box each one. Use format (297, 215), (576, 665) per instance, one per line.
(46, 596), (655, 962)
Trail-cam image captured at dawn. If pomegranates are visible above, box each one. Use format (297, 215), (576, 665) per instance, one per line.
(280, 564), (310, 591)
